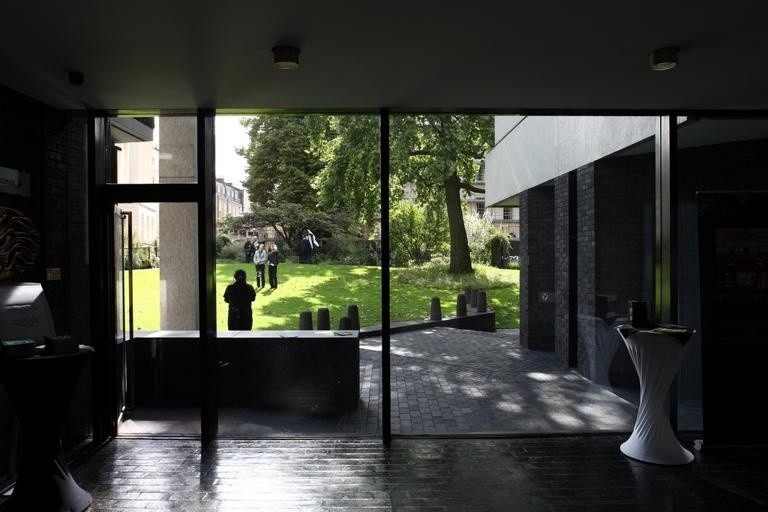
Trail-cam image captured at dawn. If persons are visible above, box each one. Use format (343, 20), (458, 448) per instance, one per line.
(244, 239), (253, 264)
(303, 229), (320, 264)
(299, 229), (311, 263)
(266, 244), (278, 290)
(252, 243), (267, 289)
(251, 235), (261, 256)
(223, 268), (257, 331)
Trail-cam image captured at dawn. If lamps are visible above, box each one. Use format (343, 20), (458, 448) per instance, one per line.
(648, 48), (678, 71)
(271, 45), (300, 71)
